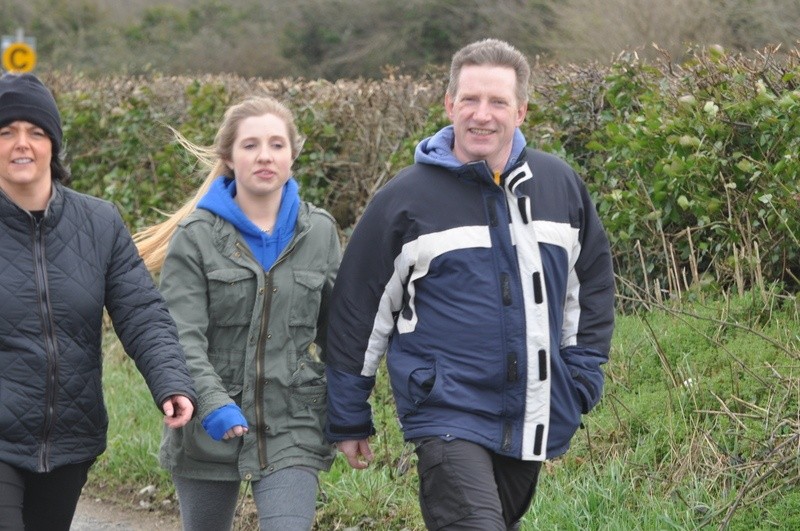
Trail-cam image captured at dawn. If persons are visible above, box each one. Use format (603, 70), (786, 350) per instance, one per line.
(326, 36), (618, 530)
(0, 74), (197, 531)
(132, 97), (344, 531)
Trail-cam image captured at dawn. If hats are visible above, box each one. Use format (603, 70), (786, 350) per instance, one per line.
(0, 72), (63, 155)
